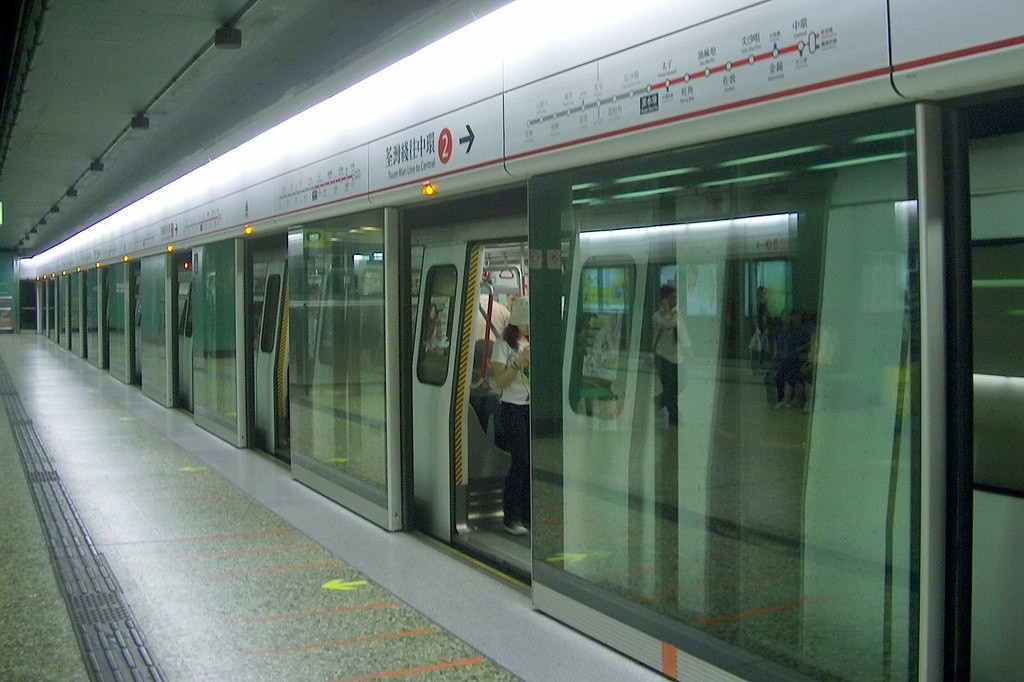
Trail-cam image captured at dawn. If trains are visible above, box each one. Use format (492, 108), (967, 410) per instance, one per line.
(20, 0), (1023, 680)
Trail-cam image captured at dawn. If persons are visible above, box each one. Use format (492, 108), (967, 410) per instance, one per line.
(303, 283), (321, 395)
(424, 305), (442, 356)
(653, 286), (678, 427)
(600, 308), (620, 369)
(369, 292), (381, 366)
(569, 312), (598, 412)
(750, 286), (817, 413)
(467, 282), (530, 534)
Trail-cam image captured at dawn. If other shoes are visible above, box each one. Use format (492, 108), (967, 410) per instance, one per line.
(766, 400), (811, 415)
(504, 523), (528, 536)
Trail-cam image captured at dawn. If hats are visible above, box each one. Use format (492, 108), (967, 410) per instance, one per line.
(508, 296), (530, 326)
